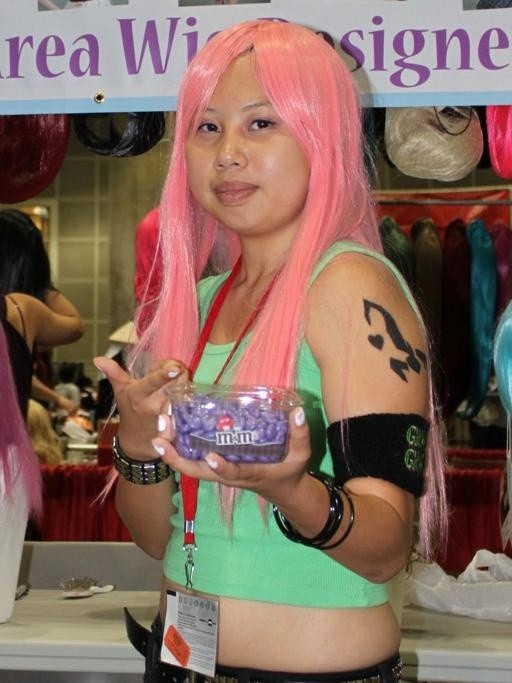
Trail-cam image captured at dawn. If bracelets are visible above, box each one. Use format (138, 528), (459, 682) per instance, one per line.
(110, 433), (172, 487)
(273, 468), (355, 551)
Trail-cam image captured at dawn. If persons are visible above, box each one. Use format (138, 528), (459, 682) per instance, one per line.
(91, 19), (448, 683)
(30, 347), (78, 416)
(51, 361), (78, 414)
(1, 288), (84, 440)
(0, 208), (60, 299)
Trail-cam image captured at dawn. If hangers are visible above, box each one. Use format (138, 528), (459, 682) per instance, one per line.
(380, 197), (508, 226)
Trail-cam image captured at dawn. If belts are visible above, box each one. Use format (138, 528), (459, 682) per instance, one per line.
(120, 605), (405, 683)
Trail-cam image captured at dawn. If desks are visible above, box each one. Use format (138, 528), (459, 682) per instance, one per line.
(2, 542), (512, 681)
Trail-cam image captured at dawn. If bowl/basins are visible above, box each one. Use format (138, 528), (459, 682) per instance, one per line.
(162, 382), (306, 464)
